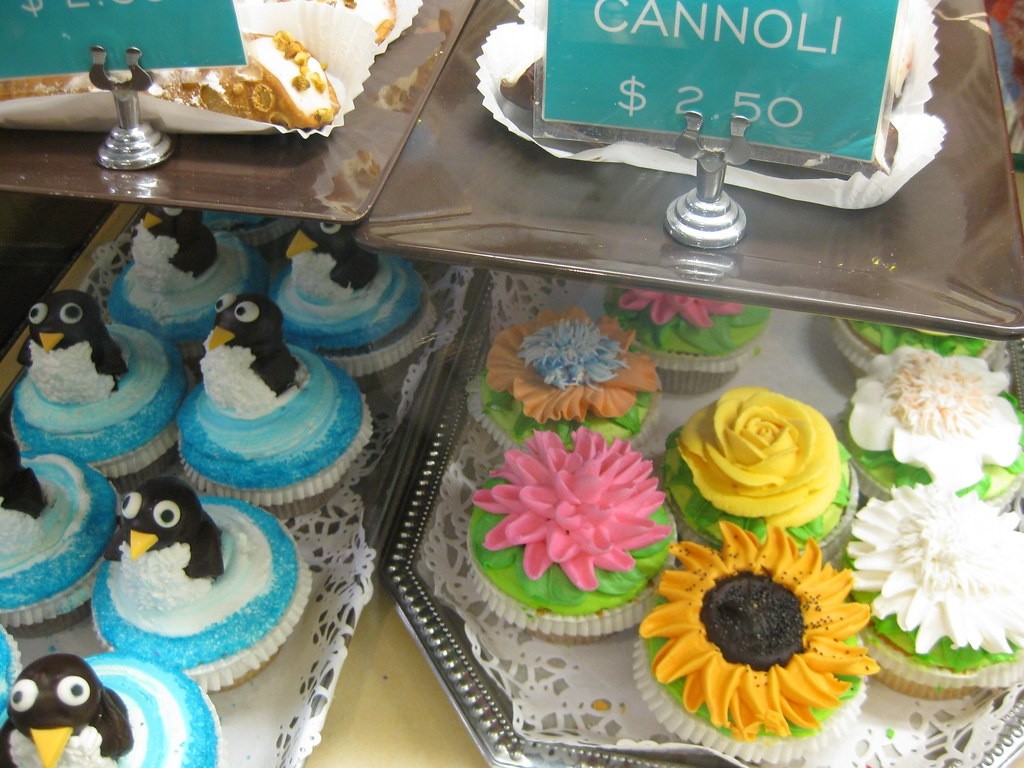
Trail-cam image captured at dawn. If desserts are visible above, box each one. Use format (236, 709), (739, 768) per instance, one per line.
(0, 207), (436, 768)
(467, 274), (1024, 768)
(0, 1), (399, 132)
